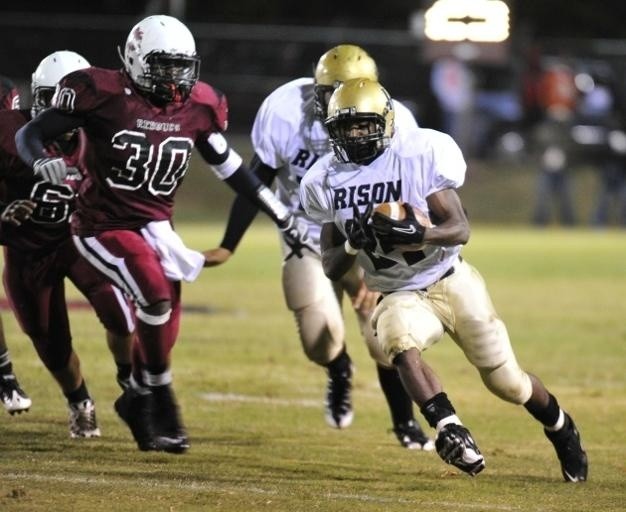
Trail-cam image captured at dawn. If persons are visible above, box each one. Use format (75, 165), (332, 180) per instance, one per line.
(297, 77), (588, 483)
(201, 46), (437, 452)
(0, 76), (33, 413)
(12, 9), (319, 455)
(1, 49), (138, 441)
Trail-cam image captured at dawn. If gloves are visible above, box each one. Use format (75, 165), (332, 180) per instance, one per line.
(32, 155), (68, 186)
(346, 201), (373, 249)
(280, 212), (322, 258)
(368, 202), (425, 249)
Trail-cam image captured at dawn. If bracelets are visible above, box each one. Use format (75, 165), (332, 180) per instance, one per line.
(344, 242), (360, 258)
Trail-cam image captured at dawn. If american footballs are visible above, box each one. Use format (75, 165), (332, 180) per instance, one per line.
(374, 202), (430, 252)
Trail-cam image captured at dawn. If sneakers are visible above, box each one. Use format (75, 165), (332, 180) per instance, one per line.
(434, 423), (485, 477)
(543, 411), (588, 482)
(0, 373), (31, 415)
(68, 398), (100, 437)
(113, 372), (190, 451)
(321, 357), (355, 429)
(393, 419), (435, 451)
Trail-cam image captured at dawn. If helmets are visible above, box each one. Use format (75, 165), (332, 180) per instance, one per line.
(124, 14), (200, 105)
(312, 45), (394, 164)
(30, 50), (92, 119)
